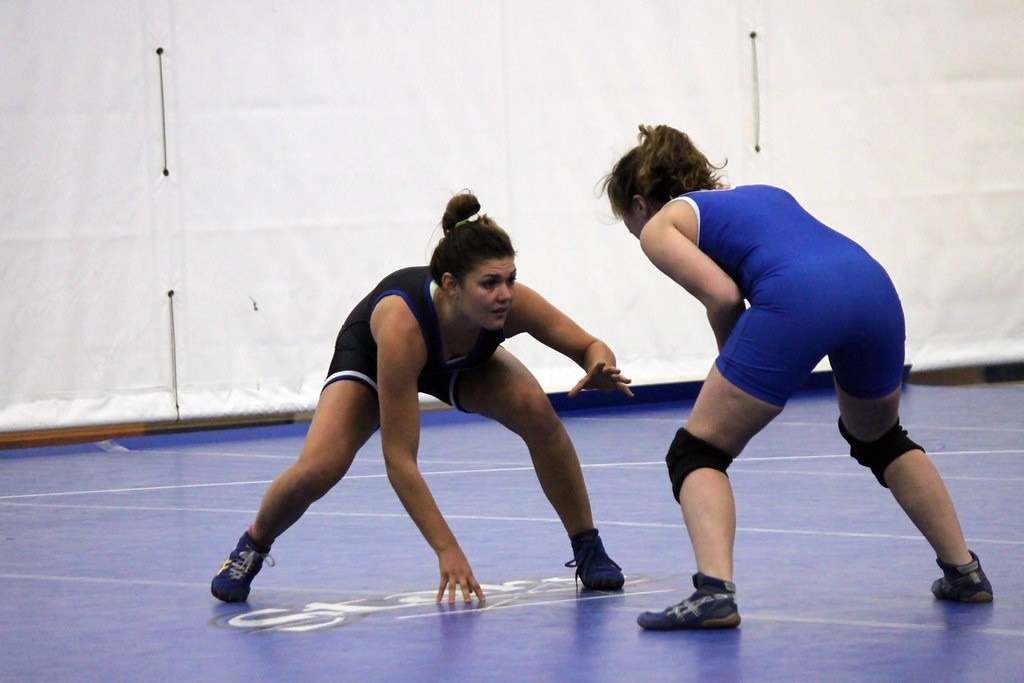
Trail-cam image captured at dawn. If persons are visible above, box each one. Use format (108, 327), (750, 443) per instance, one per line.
(594, 124), (994, 630)
(211, 193), (634, 604)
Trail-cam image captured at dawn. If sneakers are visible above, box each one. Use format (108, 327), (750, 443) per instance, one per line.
(211, 530), (274, 603)
(931, 551), (993, 603)
(637, 572), (740, 630)
(564, 527), (625, 591)
